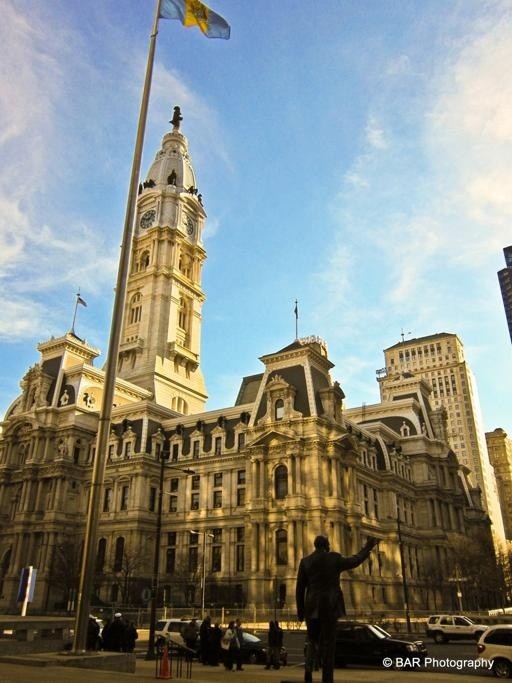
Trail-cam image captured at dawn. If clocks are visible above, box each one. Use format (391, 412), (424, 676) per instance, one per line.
(136, 206), (157, 230)
(179, 210), (197, 238)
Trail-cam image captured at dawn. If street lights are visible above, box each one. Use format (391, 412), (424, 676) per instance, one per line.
(144, 458), (197, 660)
(187, 529), (215, 617)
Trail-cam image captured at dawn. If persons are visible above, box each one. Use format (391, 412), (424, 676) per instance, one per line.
(296, 536), (378, 683)
(184, 616), (283, 670)
(87, 612), (138, 652)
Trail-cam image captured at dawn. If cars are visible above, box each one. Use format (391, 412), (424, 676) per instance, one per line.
(424, 614), (488, 644)
(302, 616), (429, 672)
(475, 623), (512, 677)
(149, 618), (288, 665)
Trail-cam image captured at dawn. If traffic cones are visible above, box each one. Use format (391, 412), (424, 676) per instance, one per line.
(159, 645), (171, 678)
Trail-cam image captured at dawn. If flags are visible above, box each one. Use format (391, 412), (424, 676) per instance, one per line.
(159, 0), (230, 39)
(77, 298), (87, 307)
